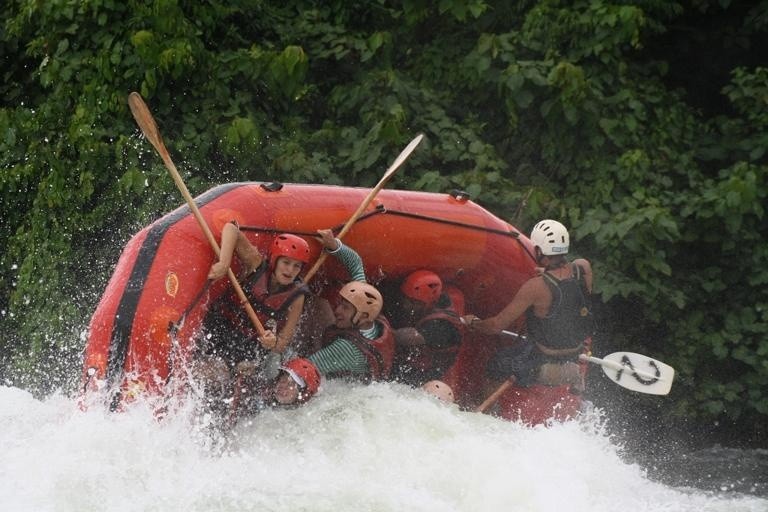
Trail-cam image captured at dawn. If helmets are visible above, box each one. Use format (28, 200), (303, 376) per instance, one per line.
(399, 270), (443, 315)
(421, 380), (455, 403)
(530, 219), (570, 262)
(274, 359), (321, 404)
(266, 234), (311, 277)
(339, 281), (384, 329)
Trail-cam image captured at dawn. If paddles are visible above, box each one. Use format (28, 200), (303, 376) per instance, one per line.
(303, 134), (424, 284)
(459, 316), (675, 395)
(128, 92), (266, 337)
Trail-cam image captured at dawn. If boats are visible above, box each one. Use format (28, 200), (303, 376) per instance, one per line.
(81, 182), (592, 431)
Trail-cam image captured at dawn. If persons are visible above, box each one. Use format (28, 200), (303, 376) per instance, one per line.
(461, 219), (595, 426)
(192, 223), (467, 431)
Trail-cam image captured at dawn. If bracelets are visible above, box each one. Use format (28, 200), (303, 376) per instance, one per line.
(471, 318), (480, 328)
